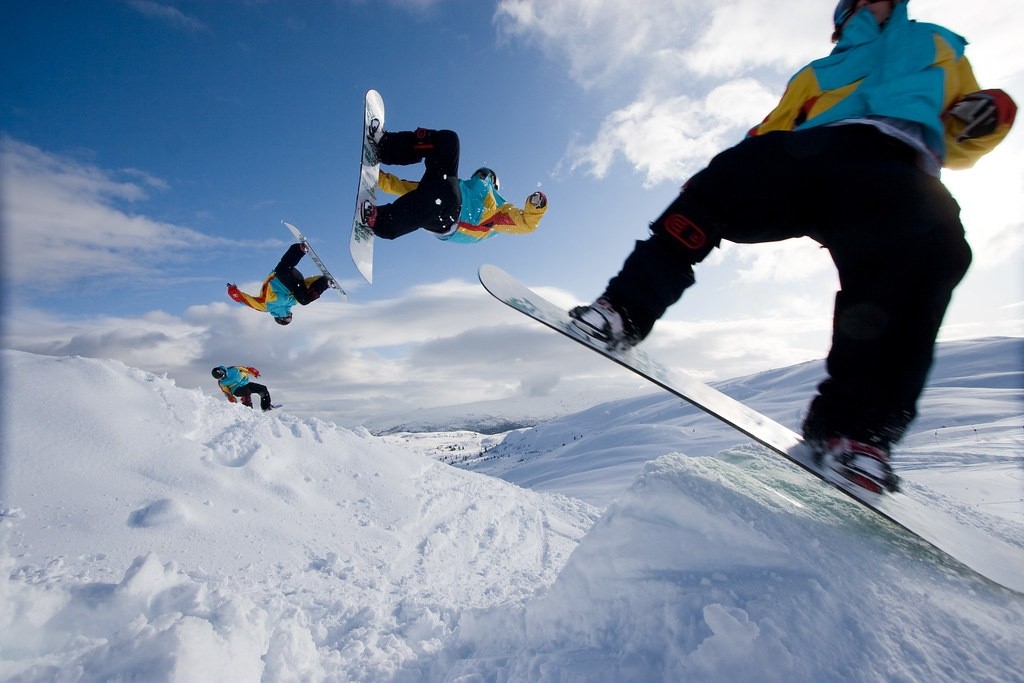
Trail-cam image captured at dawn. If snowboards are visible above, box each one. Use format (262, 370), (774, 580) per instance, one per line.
(478, 261), (1024, 596)
(261, 404), (283, 413)
(283, 221), (349, 303)
(349, 90), (386, 286)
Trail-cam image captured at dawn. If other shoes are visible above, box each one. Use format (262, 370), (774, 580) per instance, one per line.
(361, 199), (380, 230)
(565, 292), (643, 351)
(369, 118), (383, 165)
(299, 241), (310, 257)
(323, 272), (335, 293)
(806, 431), (904, 506)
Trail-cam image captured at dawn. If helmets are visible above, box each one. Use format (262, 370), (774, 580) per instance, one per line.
(274, 317), (291, 326)
(471, 167), (500, 191)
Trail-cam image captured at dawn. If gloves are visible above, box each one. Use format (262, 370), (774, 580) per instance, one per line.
(227, 282), (233, 288)
(528, 191), (545, 209)
(948, 93), (997, 143)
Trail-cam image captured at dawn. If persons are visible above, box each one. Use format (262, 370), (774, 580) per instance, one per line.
(565, 0), (1018, 492)
(361, 112), (550, 246)
(211, 364), (275, 415)
(226, 242), (336, 325)
